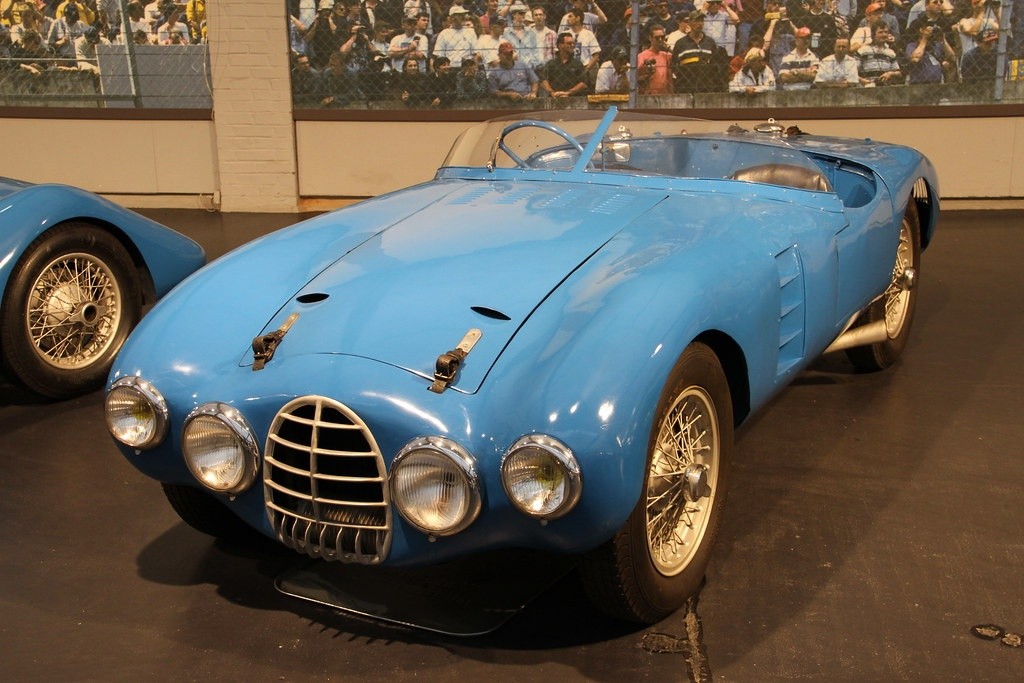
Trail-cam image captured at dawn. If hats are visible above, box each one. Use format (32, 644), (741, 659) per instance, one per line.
(449, 5), (469, 16)
(84, 26), (100, 44)
(489, 15), (508, 24)
(499, 42), (516, 54)
(509, 1), (527, 14)
(165, 4), (183, 13)
(64, 2), (80, 20)
(402, 13), (418, 23)
(624, 8), (642, 18)
(461, 55), (474, 67)
(865, 3), (884, 14)
(795, 27), (812, 37)
(612, 46), (628, 56)
(689, 10), (706, 20)
(18, 4), (33, 17)
(976, 30), (999, 43)
(317, 0), (334, 11)
(655, 0), (668, 8)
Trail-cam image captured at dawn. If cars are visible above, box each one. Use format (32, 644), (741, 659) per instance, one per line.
(0, 177), (205, 407)
(106, 106), (938, 632)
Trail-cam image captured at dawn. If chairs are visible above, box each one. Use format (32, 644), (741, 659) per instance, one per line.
(732, 162), (827, 191)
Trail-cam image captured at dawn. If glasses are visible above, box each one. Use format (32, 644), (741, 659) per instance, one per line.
(684, 19), (689, 22)
(657, 4), (668, 8)
(653, 35), (665, 39)
(518, 12), (526, 15)
(932, 0), (943, 4)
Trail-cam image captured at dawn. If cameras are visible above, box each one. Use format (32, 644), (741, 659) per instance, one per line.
(931, 27), (940, 33)
(644, 58), (656, 72)
(984, 0), (1001, 5)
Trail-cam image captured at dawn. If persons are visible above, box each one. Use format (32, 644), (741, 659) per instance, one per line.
(638, 0), (1010, 105)
(287, 0), (629, 110)
(0, 2), (206, 85)
